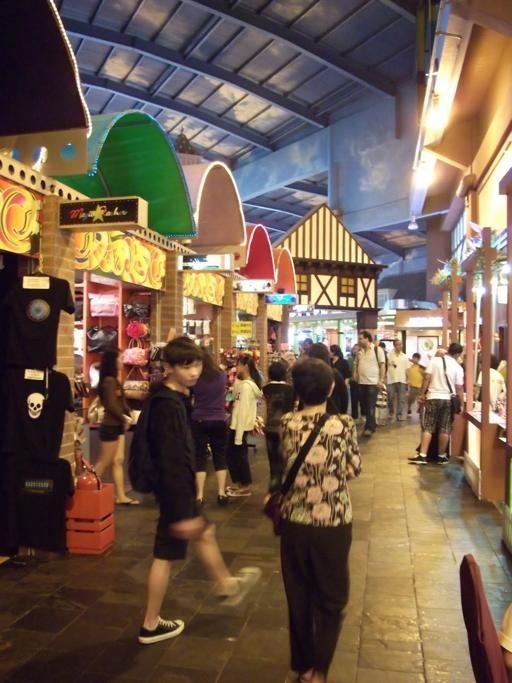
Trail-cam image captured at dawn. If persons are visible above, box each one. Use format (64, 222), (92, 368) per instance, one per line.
(261, 362), (296, 494)
(497, 602), (512, 672)
(225, 356), (263, 497)
(138, 337), (261, 644)
(278, 357), (361, 683)
(190, 346), (229, 508)
(303, 332), (508, 465)
(93, 347), (140, 505)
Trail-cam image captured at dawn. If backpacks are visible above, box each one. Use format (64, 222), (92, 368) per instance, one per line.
(129, 393), (185, 493)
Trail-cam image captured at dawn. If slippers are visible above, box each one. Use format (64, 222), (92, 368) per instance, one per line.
(116, 498), (140, 506)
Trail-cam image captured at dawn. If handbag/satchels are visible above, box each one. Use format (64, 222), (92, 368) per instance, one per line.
(88, 395), (105, 425)
(265, 489), (283, 536)
(451, 395), (460, 414)
(123, 317), (151, 399)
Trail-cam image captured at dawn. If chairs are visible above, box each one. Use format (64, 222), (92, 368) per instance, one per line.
(460, 553), (512, 683)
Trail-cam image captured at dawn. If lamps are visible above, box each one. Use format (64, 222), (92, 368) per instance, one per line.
(408, 209), (449, 229)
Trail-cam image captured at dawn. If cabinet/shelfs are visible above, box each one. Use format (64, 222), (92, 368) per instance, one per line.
(74, 270), (151, 429)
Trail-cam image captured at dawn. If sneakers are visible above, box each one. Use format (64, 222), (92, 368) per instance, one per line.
(139, 616), (185, 644)
(217, 567), (261, 605)
(408, 454), (427, 464)
(218, 481), (252, 502)
(437, 456), (449, 465)
